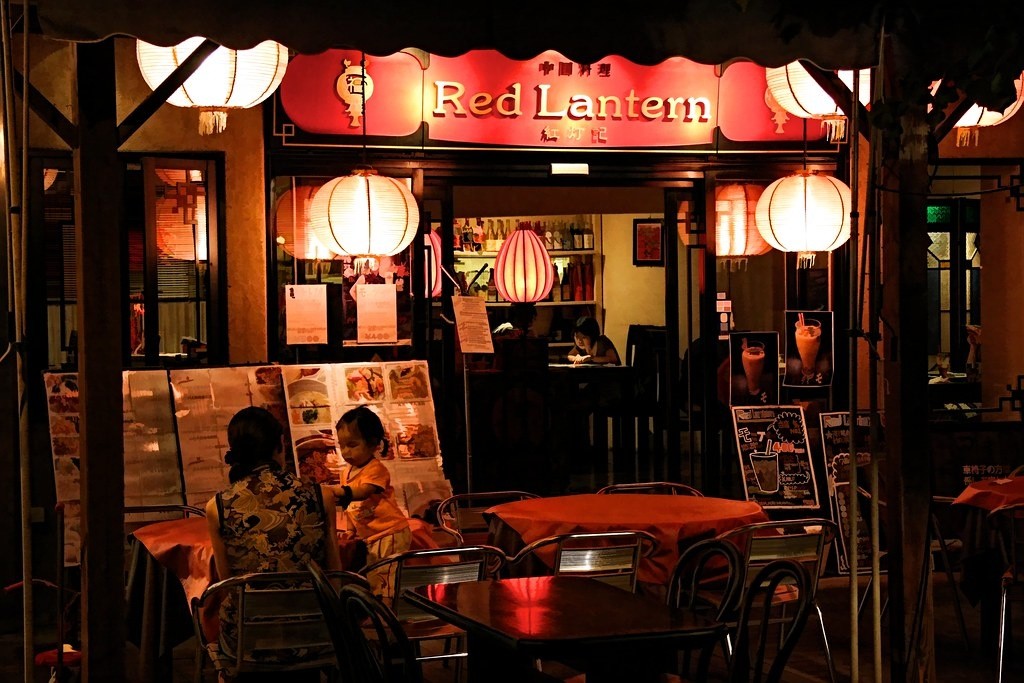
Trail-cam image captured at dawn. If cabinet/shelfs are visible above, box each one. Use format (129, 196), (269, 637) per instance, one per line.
(452, 249), (597, 347)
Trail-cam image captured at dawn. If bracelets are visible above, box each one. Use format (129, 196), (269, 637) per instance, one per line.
(342, 485), (352, 510)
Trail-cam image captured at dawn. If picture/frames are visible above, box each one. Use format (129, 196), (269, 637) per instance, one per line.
(632, 218), (665, 267)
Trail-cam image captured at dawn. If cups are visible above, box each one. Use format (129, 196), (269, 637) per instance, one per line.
(741, 341), (766, 396)
(794, 319), (822, 379)
(936, 356), (948, 377)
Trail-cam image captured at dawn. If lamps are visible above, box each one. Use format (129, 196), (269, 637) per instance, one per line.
(493, 220), (555, 336)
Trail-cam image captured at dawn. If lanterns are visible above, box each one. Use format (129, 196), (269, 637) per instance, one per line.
(677, 181), (773, 272)
(155, 168), (210, 271)
(766, 61), (871, 139)
(275, 183), (345, 274)
(136, 37), (288, 135)
(409, 226), (444, 298)
(311, 168), (420, 275)
(42, 168), (59, 190)
(754, 170), (851, 270)
(928, 71), (1024, 146)
(494, 225), (554, 302)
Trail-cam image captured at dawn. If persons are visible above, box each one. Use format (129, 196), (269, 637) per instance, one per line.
(966, 322), (982, 381)
(321, 407), (413, 628)
(681, 336), (700, 417)
(482, 305), (538, 370)
(567, 316), (623, 453)
(206, 406), (342, 683)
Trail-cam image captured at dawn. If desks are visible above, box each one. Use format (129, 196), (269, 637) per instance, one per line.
(929, 374), (982, 408)
(124, 517), (464, 683)
(481, 494), (782, 594)
(402, 576), (729, 682)
(951, 477), (1024, 673)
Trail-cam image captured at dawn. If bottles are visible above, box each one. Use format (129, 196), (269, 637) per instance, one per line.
(457, 263), (593, 302)
(453, 217), (594, 251)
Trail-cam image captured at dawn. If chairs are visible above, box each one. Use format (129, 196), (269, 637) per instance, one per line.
(120, 467), (1024, 682)
